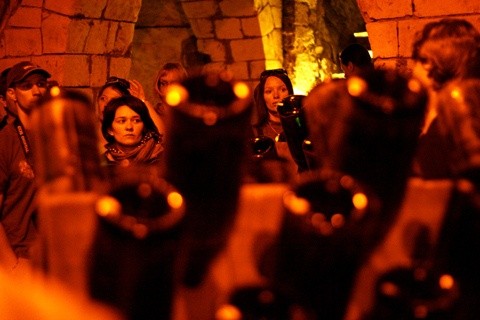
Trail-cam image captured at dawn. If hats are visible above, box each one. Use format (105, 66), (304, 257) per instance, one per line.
(7, 61), (50, 88)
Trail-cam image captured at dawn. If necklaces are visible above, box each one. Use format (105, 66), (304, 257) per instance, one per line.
(268, 122), (282, 142)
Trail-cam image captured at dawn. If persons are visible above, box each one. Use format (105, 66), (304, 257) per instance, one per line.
(410, 18), (480, 193)
(98, 95), (165, 185)
(98, 76), (164, 154)
(153, 63), (187, 123)
(340, 43), (375, 79)
(0, 62), (97, 285)
(251, 69), (302, 183)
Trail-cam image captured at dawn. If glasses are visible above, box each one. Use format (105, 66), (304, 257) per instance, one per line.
(261, 69), (288, 79)
(107, 76), (130, 88)
